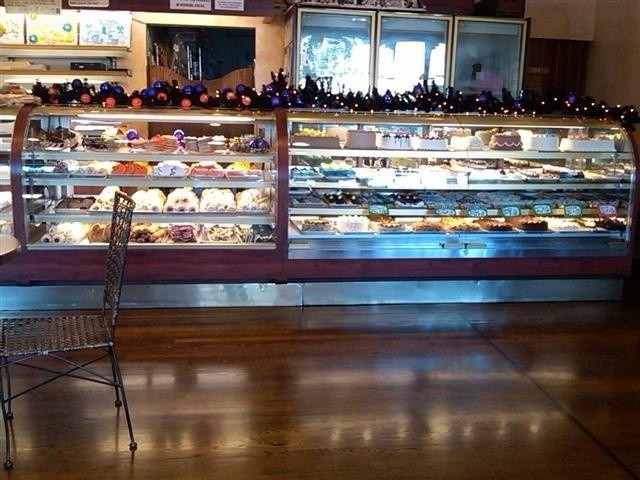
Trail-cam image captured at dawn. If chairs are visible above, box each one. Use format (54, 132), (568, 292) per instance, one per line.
(1, 191), (137, 469)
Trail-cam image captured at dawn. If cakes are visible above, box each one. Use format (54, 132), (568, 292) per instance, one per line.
(379, 134), (412, 149)
(449, 136), (484, 152)
(291, 167), (324, 181)
(411, 221), (444, 232)
(552, 222), (581, 232)
(336, 214), (369, 233)
(524, 134), (560, 153)
(477, 129), (496, 144)
(292, 126), (340, 148)
(370, 220), (407, 232)
(417, 135), (450, 151)
(320, 161), (356, 179)
(479, 221), (514, 232)
(561, 136), (617, 152)
(300, 219), (333, 231)
(488, 133), (522, 151)
(517, 217), (549, 231)
(447, 221), (480, 231)
(470, 165), (587, 184)
(345, 129), (376, 149)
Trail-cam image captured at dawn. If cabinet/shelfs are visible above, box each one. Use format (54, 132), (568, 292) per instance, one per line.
(0, 104), (633, 308)
(1, 44), (133, 83)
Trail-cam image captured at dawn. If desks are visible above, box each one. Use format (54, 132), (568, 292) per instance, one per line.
(1, 234), (23, 264)
